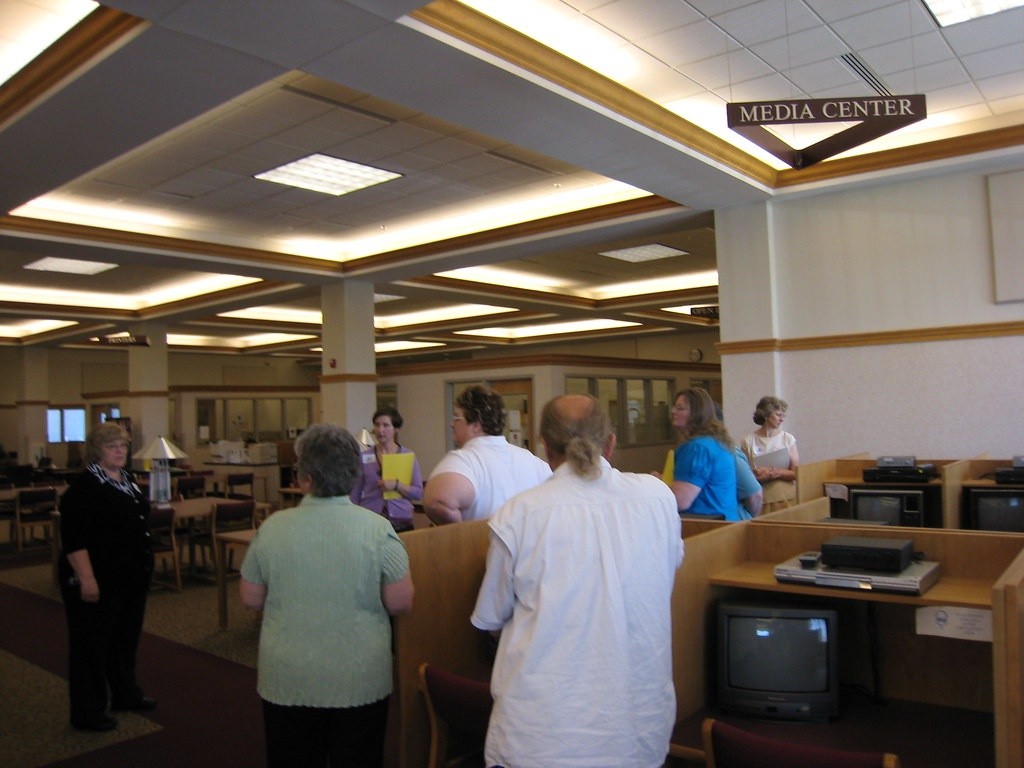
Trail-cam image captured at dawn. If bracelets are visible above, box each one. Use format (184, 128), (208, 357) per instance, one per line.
(395, 479), (399, 490)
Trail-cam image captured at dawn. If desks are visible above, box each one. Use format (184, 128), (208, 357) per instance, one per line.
(0, 484), (70, 545)
(142, 497), (247, 589)
(373, 458), (1024, 768)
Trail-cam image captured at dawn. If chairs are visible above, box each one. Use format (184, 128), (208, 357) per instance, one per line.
(0, 455), (303, 632)
(417, 662), (493, 767)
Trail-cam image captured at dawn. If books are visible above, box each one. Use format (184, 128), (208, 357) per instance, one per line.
(381, 453), (415, 500)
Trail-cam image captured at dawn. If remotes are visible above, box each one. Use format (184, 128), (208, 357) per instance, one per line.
(799, 551), (822, 565)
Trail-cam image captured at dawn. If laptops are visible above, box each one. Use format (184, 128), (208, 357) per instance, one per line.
(774, 552), (941, 594)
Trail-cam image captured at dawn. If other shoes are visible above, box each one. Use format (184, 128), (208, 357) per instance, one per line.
(72, 716), (118, 733)
(111, 695), (158, 714)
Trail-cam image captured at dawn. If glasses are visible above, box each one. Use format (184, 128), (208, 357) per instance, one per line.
(451, 414), (466, 425)
(100, 441), (130, 453)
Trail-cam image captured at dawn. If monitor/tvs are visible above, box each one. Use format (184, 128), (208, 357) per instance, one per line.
(971, 490), (1024, 534)
(714, 592), (840, 724)
(851, 489), (925, 529)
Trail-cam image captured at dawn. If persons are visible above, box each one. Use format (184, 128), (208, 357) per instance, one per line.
(651, 389), (751, 523)
(242, 426), (415, 768)
(420, 386), (553, 526)
(469, 393), (685, 768)
(55, 424), (156, 730)
(714, 402), (762, 517)
(741, 395), (799, 514)
(351, 407), (424, 533)
